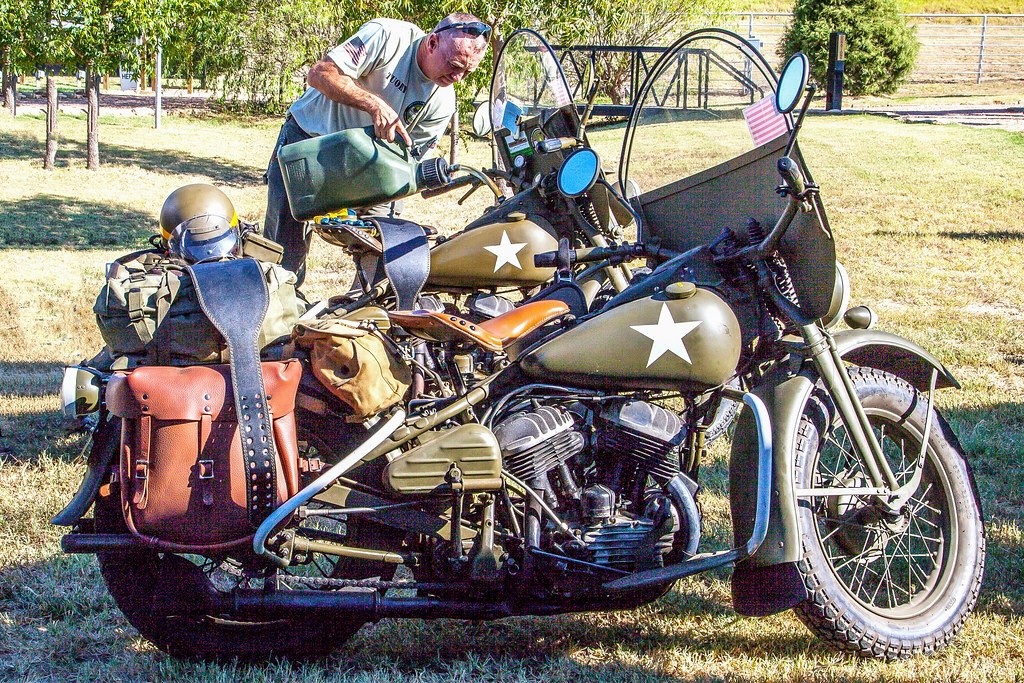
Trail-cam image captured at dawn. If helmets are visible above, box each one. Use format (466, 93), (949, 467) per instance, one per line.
(160, 184), (240, 261)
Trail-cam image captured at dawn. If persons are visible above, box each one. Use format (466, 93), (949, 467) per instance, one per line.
(262, 10), (492, 289)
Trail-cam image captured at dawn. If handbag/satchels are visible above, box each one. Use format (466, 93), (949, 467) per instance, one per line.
(103, 359), (303, 554)
(296, 295), (412, 420)
(94, 247), (297, 361)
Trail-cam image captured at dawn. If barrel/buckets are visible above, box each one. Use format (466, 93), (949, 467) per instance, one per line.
(274, 117), (460, 220)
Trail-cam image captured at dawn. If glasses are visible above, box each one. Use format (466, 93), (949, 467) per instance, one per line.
(434, 22), (492, 43)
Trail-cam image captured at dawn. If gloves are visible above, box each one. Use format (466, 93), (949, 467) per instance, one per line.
(314, 208), (379, 237)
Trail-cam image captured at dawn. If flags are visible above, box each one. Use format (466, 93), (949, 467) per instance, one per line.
(742, 92), (793, 147)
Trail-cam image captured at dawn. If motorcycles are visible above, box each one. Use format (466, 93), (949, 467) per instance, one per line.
(50, 49), (989, 672)
(285, 27), (836, 470)
